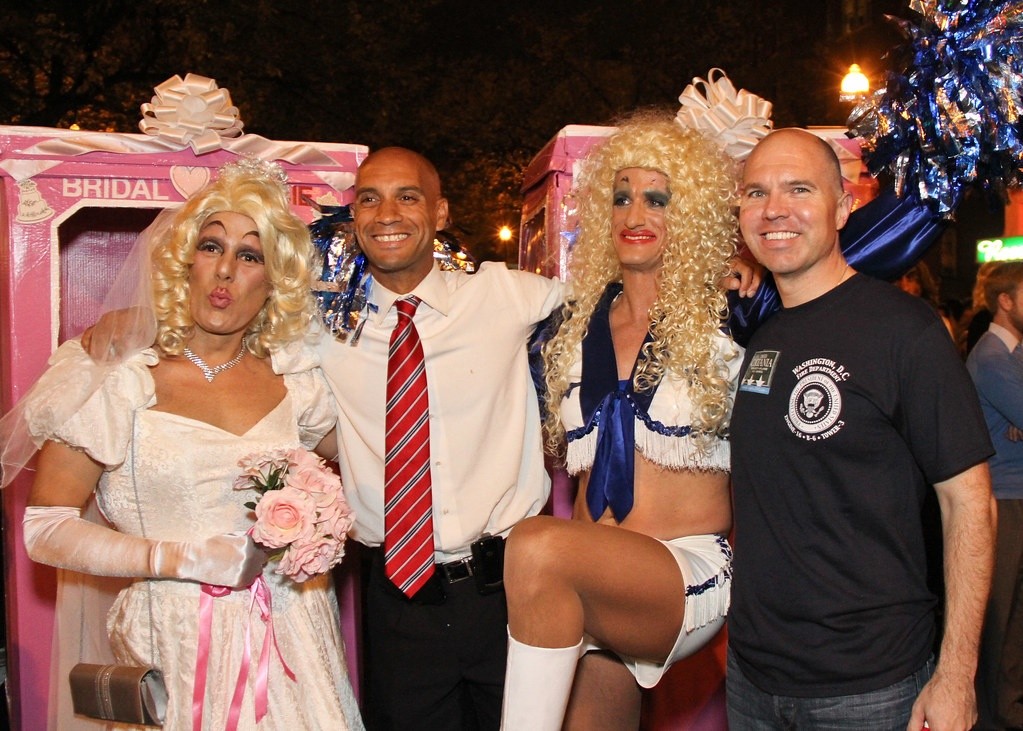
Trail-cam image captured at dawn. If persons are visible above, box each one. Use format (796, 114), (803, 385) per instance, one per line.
(959, 262), (1023, 730)
(20, 172), (366, 730)
(720, 127), (996, 731)
(496, 114), (761, 731)
(898, 264), (1002, 359)
(81, 144), (765, 730)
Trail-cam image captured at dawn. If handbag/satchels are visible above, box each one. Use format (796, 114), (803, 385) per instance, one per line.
(69, 663), (167, 728)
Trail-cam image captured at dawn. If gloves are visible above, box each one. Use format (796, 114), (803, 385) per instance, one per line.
(23, 505), (268, 591)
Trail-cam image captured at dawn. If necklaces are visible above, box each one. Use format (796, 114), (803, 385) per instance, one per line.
(181, 337), (248, 384)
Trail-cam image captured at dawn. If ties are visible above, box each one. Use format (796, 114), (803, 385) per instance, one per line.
(384, 297), (434, 597)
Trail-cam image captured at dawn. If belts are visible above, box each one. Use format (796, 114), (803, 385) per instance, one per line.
(436, 557), (475, 585)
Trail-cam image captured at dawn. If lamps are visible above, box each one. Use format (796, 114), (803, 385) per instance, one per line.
(840, 34), (870, 93)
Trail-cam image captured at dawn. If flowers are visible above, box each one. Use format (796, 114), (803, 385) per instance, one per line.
(234, 445), (358, 583)
(139, 71), (246, 158)
(672, 66), (776, 169)
